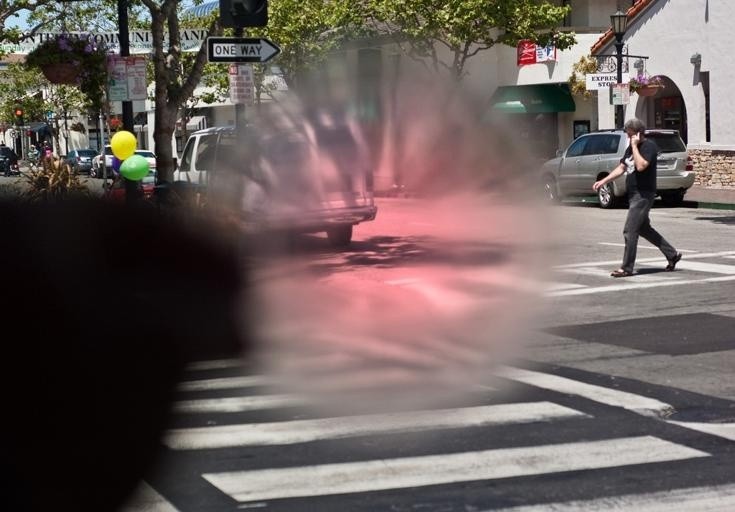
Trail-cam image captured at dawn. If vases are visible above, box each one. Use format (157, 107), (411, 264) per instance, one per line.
(42, 60), (79, 85)
(636, 84), (662, 96)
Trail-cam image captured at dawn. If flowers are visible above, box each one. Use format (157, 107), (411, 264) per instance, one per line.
(630, 72), (663, 90)
(19, 32), (114, 95)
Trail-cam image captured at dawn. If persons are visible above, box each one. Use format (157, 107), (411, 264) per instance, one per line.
(593, 117), (682, 277)
(28, 141), (52, 166)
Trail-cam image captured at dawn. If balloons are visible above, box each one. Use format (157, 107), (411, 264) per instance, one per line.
(111, 156), (124, 173)
(119, 154), (149, 181)
(111, 131), (136, 160)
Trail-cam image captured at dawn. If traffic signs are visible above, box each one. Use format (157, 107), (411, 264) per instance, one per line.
(206, 35), (283, 64)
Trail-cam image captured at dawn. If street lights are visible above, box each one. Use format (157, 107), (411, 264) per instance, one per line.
(607, 5), (630, 127)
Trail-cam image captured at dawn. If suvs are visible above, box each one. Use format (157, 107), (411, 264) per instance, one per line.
(539, 125), (694, 204)
(0, 146), (19, 170)
(90, 144), (117, 178)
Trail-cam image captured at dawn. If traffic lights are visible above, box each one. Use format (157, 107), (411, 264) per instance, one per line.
(15, 107), (24, 127)
(220, 0), (268, 26)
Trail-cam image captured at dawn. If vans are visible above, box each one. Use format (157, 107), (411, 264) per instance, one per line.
(169, 119), (380, 249)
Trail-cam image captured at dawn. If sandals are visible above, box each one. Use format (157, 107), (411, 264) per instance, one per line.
(609, 268), (636, 278)
(664, 251), (683, 272)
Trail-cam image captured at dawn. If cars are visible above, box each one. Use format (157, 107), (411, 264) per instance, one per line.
(64, 147), (100, 175)
(99, 175), (157, 202)
(131, 150), (158, 177)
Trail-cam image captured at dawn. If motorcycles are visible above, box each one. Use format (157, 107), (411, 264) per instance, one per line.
(5, 158), (20, 177)
